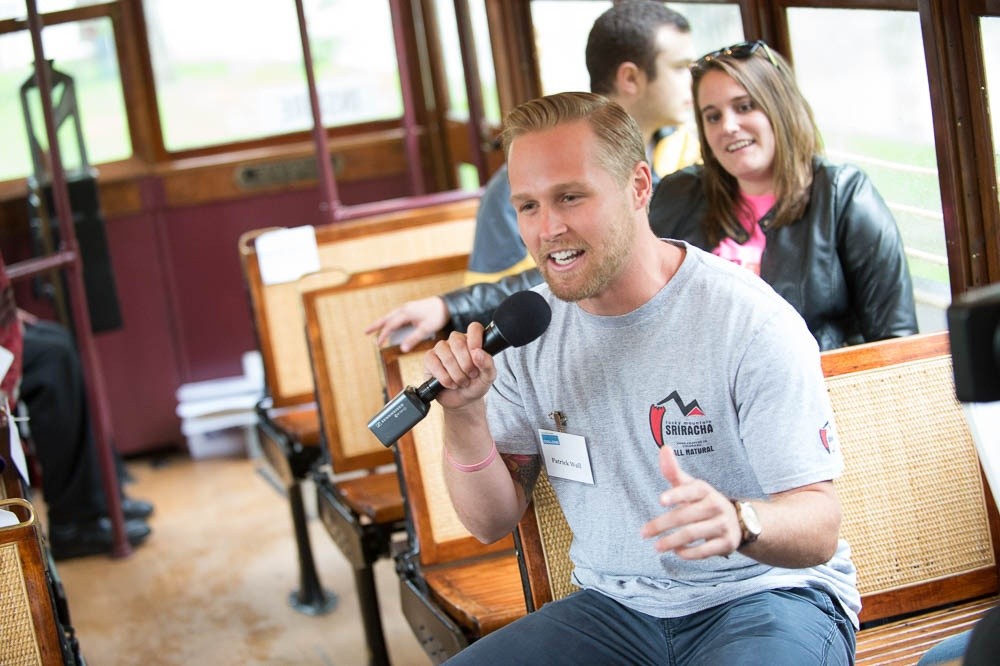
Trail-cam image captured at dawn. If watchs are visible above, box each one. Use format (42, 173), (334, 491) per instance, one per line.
(724, 498), (763, 560)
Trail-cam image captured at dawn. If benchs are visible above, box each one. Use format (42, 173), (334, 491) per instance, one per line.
(301, 244), (547, 665)
(235, 189), (557, 618)
(375, 328), (534, 666)
(513, 330), (1000, 665)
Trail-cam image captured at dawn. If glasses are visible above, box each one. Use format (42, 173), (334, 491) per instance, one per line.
(690, 40), (778, 69)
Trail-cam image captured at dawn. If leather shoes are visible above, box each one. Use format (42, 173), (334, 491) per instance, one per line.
(48, 517), (151, 560)
(107, 496), (153, 519)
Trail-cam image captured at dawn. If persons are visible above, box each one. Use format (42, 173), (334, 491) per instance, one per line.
(425, 92), (862, 666)
(18, 308), (154, 561)
(463, 0), (704, 289)
(367, 39), (920, 354)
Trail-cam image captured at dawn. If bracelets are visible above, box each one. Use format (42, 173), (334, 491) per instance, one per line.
(445, 438), (497, 471)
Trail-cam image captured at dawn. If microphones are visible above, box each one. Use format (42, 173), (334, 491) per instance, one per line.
(368, 290), (551, 448)
